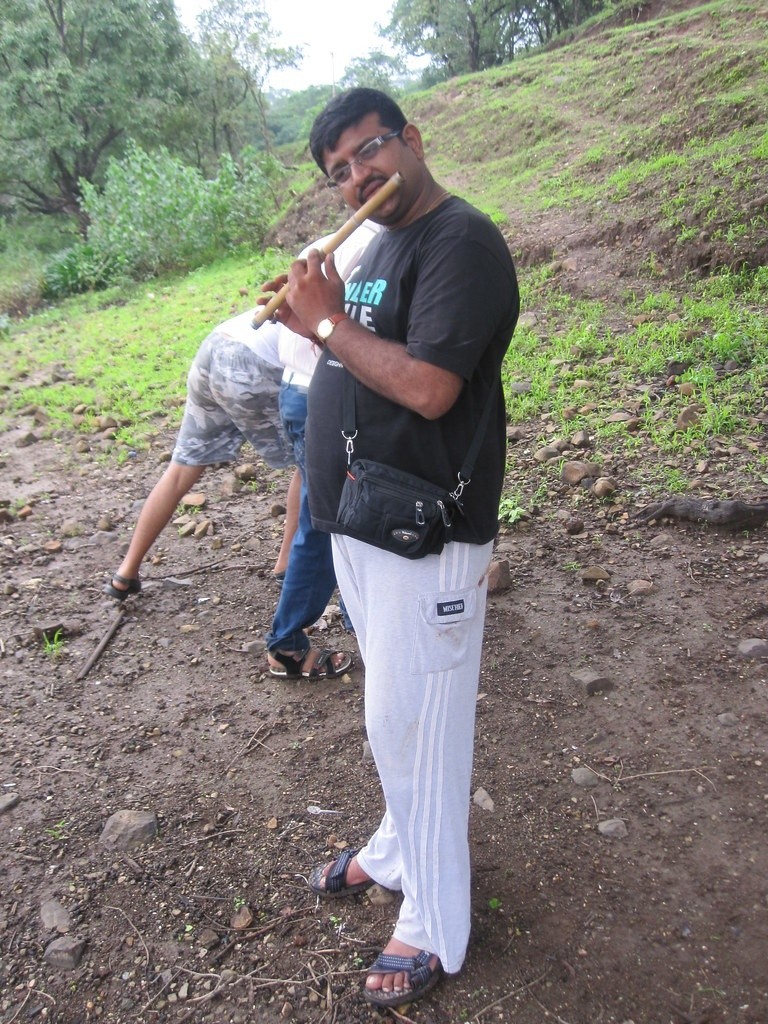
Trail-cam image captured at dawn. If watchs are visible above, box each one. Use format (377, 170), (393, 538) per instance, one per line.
(315, 312), (350, 347)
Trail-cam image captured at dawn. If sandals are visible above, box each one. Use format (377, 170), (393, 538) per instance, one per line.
(268, 641), (350, 676)
(275, 566), (287, 585)
(104, 572), (141, 599)
(311, 848), (380, 895)
(363, 936), (447, 1002)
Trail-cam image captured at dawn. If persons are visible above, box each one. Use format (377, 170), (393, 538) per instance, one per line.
(254, 86), (521, 1012)
(263, 217), (390, 680)
(106, 304), (302, 602)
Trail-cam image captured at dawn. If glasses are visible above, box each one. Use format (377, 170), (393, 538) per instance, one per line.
(326, 129), (401, 191)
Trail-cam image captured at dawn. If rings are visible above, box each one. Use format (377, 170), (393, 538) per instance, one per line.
(269, 312), (278, 325)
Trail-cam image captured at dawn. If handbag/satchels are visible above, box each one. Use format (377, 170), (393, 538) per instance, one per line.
(336, 458), (456, 562)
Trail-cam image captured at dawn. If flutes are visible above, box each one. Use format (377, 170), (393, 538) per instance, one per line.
(251, 171), (406, 330)
